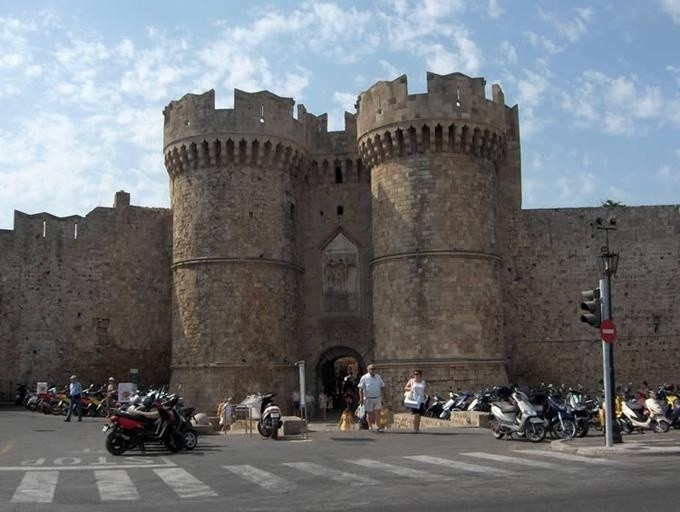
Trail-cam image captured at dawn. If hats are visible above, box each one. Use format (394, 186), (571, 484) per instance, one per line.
(70, 375), (77, 380)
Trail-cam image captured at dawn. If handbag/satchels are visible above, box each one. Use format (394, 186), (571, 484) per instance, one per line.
(404, 394), (421, 409)
(404, 390), (412, 397)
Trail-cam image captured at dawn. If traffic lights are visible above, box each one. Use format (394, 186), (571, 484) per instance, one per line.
(580, 286), (599, 327)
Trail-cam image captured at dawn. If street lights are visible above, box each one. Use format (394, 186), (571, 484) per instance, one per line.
(593, 215), (621, 444)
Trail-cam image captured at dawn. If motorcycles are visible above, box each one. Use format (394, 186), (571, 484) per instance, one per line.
(257, 391), (283, 440)
(419, 380), (680, 445)
(12, 381), (205, 456)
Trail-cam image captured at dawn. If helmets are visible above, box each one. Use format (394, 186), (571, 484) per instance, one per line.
(133, 395), (152, 406)
(663, 383), (675, 391)
(109, 377), (115, 383)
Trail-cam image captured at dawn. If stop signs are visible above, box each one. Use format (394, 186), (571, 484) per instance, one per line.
(599, 320), (617, 344)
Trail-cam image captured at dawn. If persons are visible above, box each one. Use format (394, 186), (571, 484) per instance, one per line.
(403, 370), (435, 433)
(614, 380), (680, 401)
(87, 378), (94, 389)
(213, 397), (238, 433)
(289, 360), (388, 433)
(63, 375), (83, 422)
(102, 376), (117, 418)
